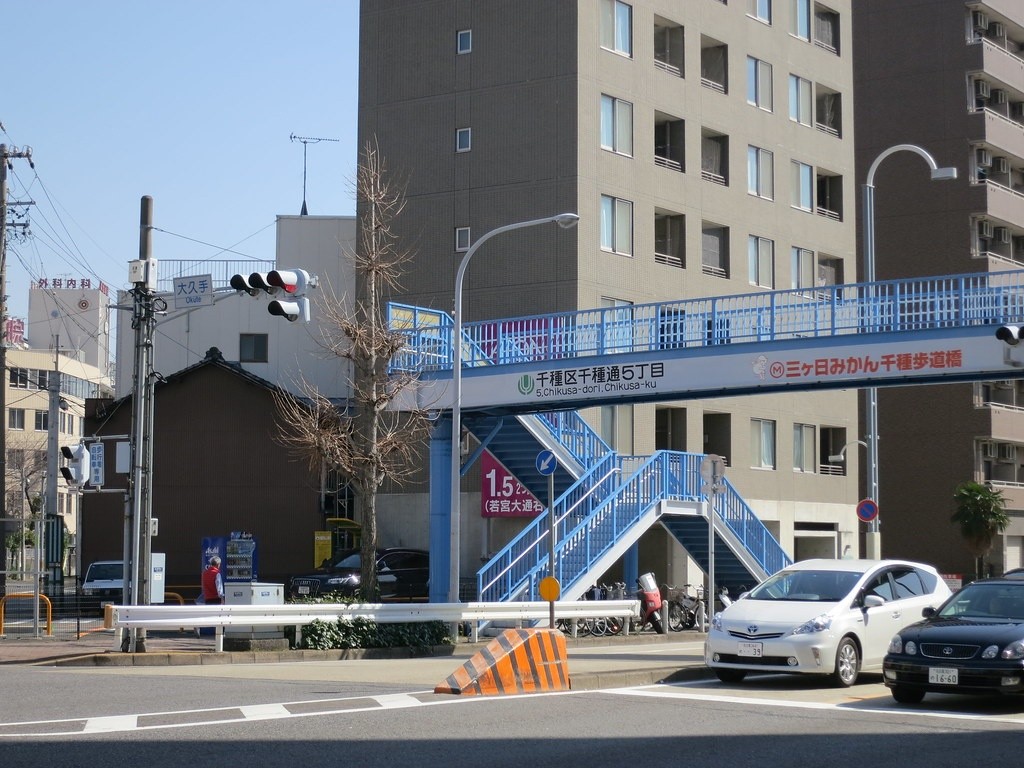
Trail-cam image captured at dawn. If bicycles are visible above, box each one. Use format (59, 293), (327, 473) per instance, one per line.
(557, 572), (757, 639)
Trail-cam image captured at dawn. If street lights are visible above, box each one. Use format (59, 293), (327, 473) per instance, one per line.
(446, 213), (581, 640)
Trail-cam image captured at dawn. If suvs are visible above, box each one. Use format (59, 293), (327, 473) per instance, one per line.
(286, 546), (430, 600)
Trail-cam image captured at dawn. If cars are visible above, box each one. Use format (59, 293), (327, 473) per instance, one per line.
(79, 560), (132, 612)
(704, 557), (958, 690)
(882, 569), (1024, 708)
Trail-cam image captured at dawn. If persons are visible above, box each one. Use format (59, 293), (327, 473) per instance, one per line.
(201, 556), (224, 605)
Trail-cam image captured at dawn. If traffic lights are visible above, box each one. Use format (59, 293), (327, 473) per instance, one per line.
(59, 444), (92, 487)
(994, 325), (1024, 369)
(230, 268), (311, 326)
